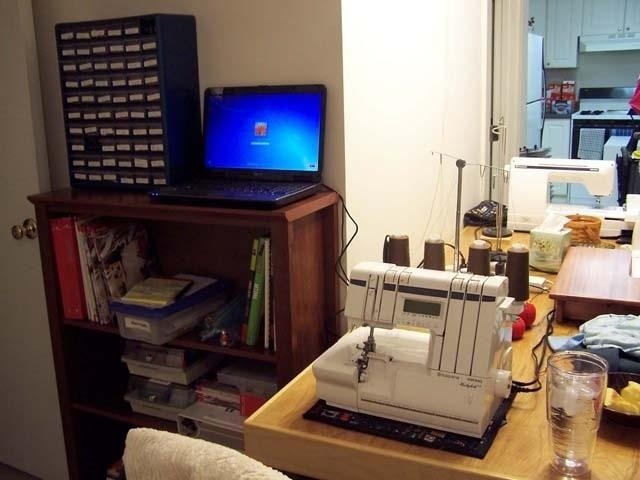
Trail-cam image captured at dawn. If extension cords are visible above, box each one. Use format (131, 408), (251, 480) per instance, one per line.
(445, 262), (548, 294)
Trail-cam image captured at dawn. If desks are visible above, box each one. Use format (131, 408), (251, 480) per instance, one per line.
(240, 267), (640, 480)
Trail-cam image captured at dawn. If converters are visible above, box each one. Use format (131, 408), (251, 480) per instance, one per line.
(494, 261), (506, 276)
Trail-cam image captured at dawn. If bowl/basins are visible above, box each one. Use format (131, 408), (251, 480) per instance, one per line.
(603, 371), (640, 429)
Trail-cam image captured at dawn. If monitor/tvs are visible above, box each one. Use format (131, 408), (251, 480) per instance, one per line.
(147, 84), (327, 209)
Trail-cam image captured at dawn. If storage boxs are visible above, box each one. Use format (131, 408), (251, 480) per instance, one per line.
(112, 272), (227, 345)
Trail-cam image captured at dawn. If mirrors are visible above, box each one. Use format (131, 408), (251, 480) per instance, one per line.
(491, 0), (640, 268)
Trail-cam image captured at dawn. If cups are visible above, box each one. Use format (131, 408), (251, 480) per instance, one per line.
(545, 349), (609, 478)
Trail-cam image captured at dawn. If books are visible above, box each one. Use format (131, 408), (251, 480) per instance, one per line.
(75, 220), (278, 355)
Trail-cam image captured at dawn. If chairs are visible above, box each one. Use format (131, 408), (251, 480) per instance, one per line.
(122, 424), (290, 480)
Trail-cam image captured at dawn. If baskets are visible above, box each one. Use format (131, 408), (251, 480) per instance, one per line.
(564, 214), (602, 246)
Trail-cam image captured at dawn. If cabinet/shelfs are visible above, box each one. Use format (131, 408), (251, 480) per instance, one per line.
(541, 119), (571, 196)
(26, 188), (339, 480)
(528, 0), (578, 69)
(55, 12), (202, 190)
(576, 0), (639, 52)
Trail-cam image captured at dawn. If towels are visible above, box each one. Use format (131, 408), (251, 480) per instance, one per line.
(611, 128), (633, 148)
(577, 128), (605, 159)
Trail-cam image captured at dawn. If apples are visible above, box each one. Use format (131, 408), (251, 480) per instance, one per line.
(519, 303), (536, 327)
(512, 316), (525, 340)
